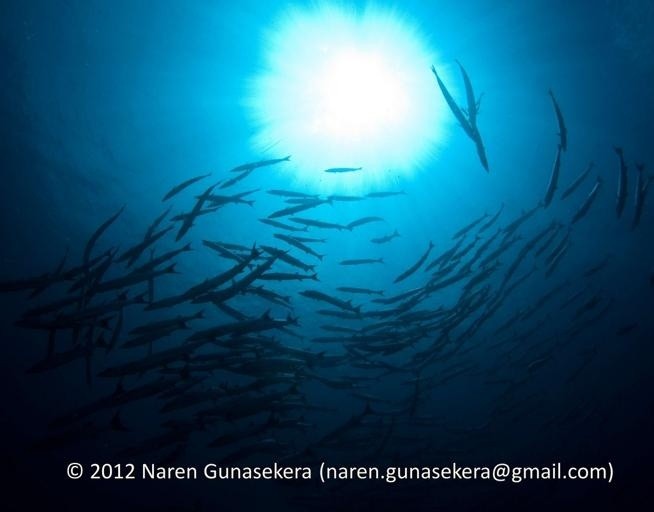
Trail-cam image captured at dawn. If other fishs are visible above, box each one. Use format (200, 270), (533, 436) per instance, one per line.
(0, 143), (654, 512)
